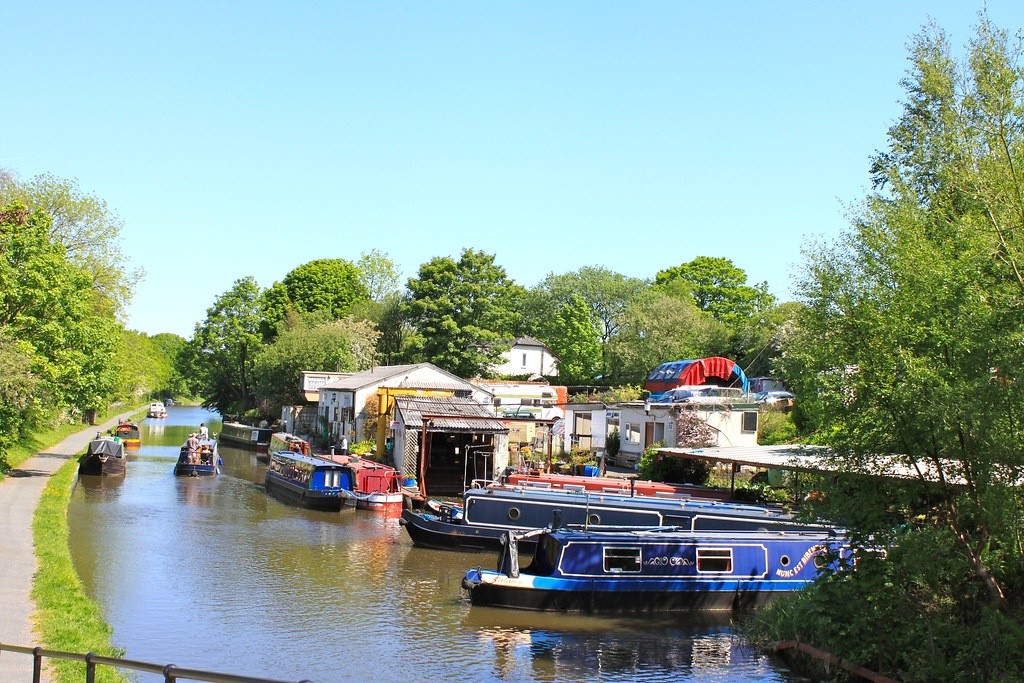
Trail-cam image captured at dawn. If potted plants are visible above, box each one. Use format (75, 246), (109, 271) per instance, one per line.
(402, 473), (417, 487)
(519, 442), (599, 476)
(299, 415), (341, 455)
(604, 427), (621, 467)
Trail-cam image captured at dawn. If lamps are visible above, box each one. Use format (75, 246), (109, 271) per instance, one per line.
(645, 402), (650, 416)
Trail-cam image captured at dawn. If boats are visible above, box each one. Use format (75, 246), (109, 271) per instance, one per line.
(313, 446), (404, 511)
(148, 402), (168, 418)
(268, 431), (311, 459)
(116, 419), (142, 449)
(175, 432), (222, 477)
(264, 450), (359, 513)
(461, 493), (898, 617)
(219, 422), (271, 450)
(397, 473), (836, 551)
(85, 432), (127, 477)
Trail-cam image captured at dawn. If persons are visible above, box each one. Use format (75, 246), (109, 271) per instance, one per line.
(200, 423), (208, 440)
(187, 433), (202, 463)
(340, 436), (348, 455)
(293, 444), (303, 454)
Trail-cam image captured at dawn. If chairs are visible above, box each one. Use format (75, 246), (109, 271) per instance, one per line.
(439, 505), (452, 524)
